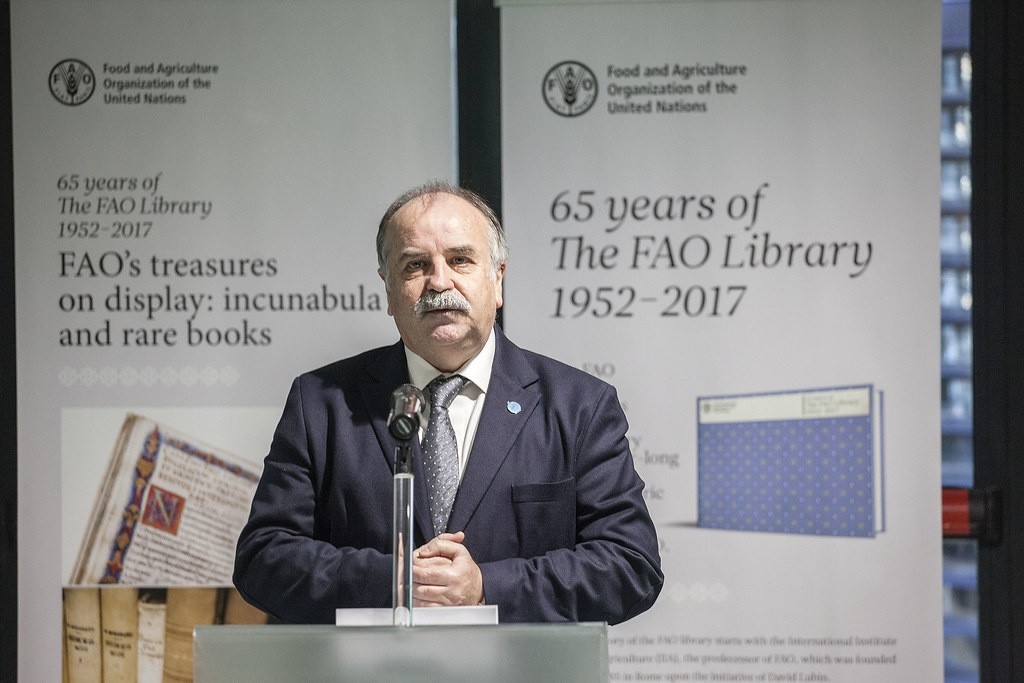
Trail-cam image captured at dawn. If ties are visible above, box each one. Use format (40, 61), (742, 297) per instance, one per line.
(422, 375), (470, 538)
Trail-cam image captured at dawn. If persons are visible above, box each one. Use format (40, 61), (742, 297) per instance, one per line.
(231, 181), (665, 626)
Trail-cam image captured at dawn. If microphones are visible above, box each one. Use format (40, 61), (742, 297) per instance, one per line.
(388, 383), (427, 442)
